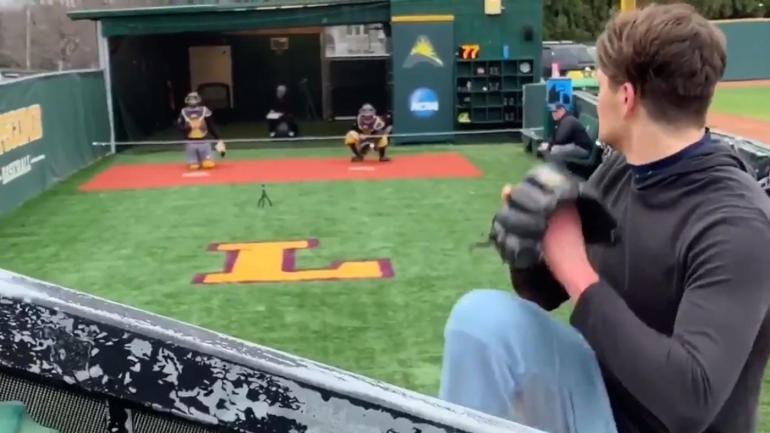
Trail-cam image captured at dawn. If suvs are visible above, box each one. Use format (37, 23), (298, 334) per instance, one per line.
(542, 41), (595, 76)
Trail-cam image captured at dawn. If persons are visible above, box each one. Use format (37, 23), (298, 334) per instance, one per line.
(178, 92), (225, 169)
(345, 105), (393, 162)
(267, 85), (296, 138)
(537, 103), (595, 172)
(441, 4), (770, 433)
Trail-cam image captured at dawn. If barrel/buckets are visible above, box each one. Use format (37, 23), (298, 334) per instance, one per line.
(546, 77), (573, 113)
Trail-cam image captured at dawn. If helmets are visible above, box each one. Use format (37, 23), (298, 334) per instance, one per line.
(184, 92), (201, 105)
(360, 104), (376, 125)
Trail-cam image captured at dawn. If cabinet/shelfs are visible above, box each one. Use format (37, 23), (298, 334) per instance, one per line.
(454, 57), (536, 128)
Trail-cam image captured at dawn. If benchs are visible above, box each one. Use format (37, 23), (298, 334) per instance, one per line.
(567, 112), (601, 167)
(520, 126), (544, 158)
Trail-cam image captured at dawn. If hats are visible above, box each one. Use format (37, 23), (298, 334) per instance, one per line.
(551, 102), (565, 112)
(277, 86), (286, 91)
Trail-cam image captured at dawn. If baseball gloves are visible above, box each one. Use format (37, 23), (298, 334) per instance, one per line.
(489, 162), (619, 311)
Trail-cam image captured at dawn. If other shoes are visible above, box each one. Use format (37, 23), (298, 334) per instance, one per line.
(270, 132), (275, 137)
(352, 155), (363, 162)
(288, 132), (294, 137)
(379, 156), (391, 161)
